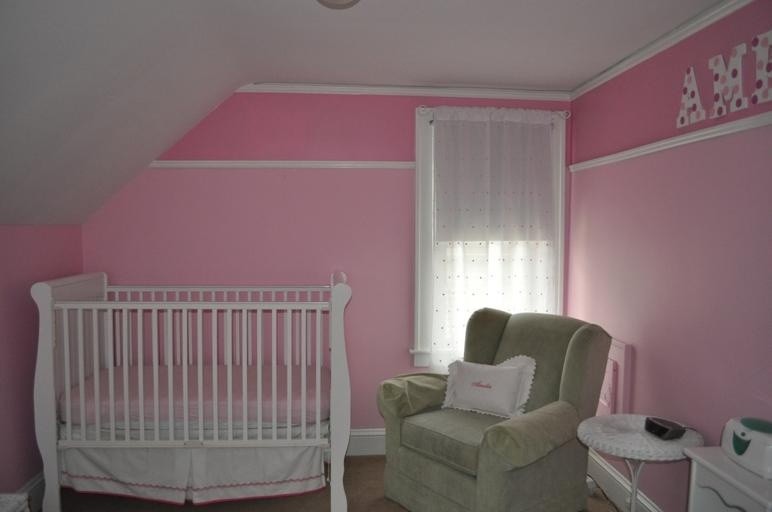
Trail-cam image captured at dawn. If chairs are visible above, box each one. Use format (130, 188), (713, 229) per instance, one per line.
(377, 308), (612, 512)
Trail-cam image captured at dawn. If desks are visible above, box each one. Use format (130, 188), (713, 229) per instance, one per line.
(682, 446), (772, 512)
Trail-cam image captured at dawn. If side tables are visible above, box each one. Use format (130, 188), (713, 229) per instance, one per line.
(577, 413), (704, 512)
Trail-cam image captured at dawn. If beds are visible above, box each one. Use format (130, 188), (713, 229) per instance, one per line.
(30, 270), (353, 512)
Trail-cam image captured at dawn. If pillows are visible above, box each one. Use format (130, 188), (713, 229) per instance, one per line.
(441, 354), (536, 418)
